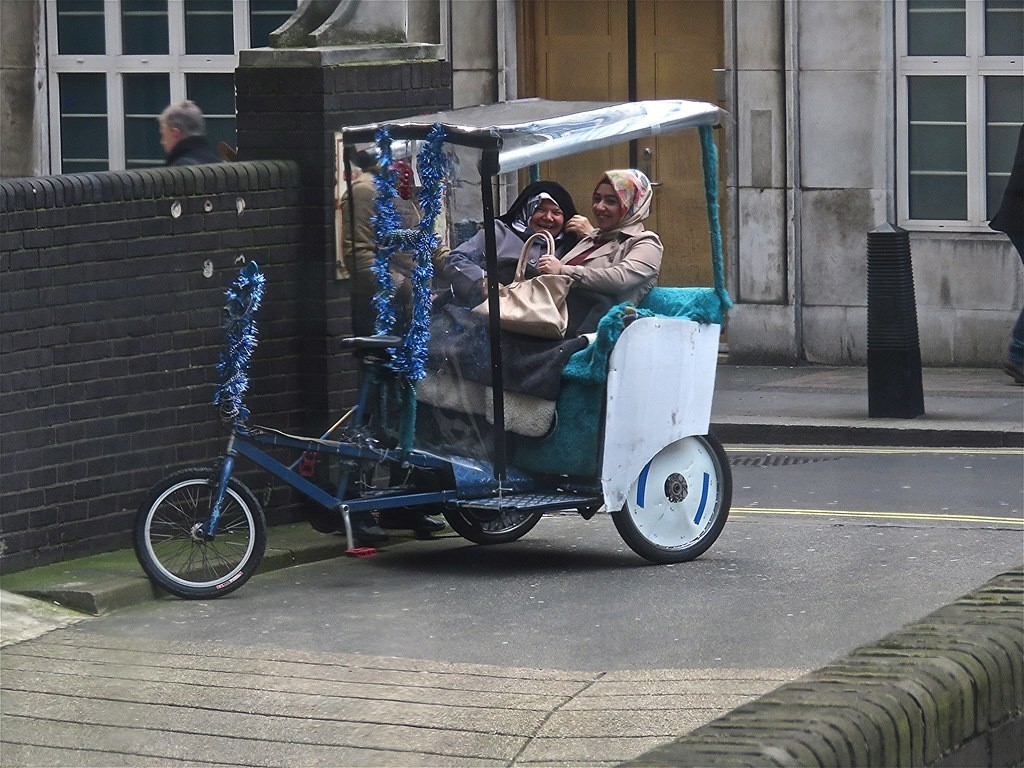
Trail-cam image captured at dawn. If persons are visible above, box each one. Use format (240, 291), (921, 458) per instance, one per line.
(339, 158), (665, 530)
(158, 100), (225, 166)
(987, 121), (1024, 383)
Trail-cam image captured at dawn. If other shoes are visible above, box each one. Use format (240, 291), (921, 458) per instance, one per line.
(1004, 359), (1024, 383)
(338, 525), (388, 542)
(378, 508), (445, 533)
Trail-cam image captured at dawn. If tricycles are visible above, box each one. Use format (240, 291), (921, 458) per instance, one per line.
(133, 100), (739, 603)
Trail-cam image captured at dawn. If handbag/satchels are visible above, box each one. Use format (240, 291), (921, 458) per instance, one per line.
(471, 228), (573, 341)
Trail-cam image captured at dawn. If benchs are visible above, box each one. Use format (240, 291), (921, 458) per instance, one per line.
(414, 287), (727, 482)
